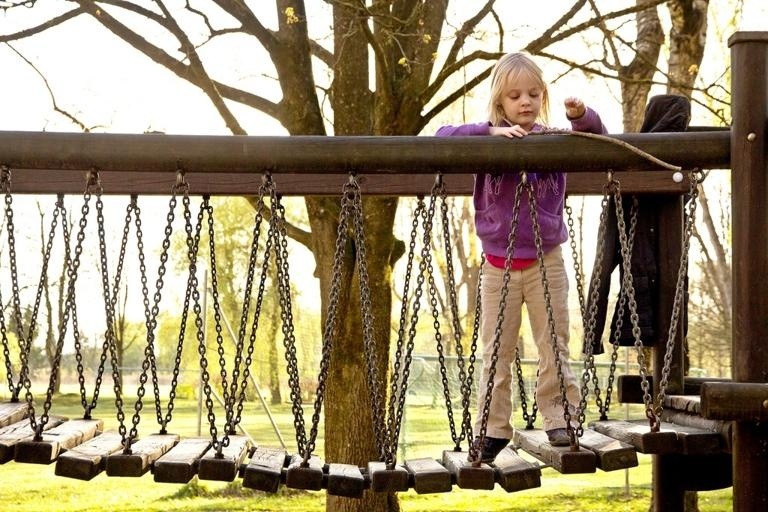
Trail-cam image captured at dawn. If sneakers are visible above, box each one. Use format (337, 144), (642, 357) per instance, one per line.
(546, 428), (575, 446)
(468, 436), (510, 463)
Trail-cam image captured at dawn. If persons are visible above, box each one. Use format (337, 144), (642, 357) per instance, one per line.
(427, 49), (610, 466)
